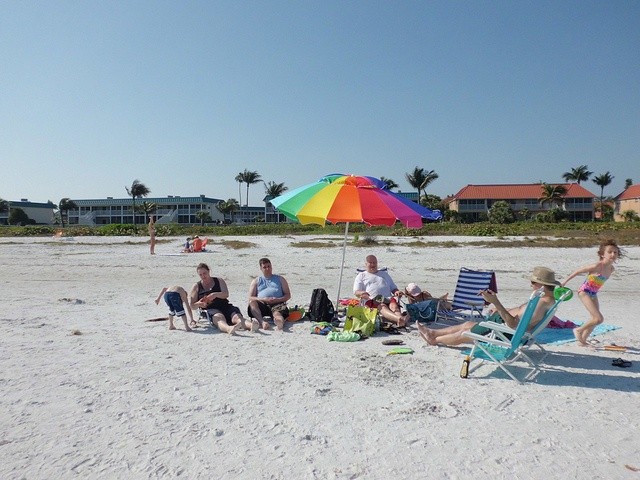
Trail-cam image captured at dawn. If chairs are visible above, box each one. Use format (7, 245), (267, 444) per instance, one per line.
(196, 308), (214, 328)
(480, 289), (572, 366)
(356, 266), (398, 328)
(435, 267), (494, 327)
(461, 285), (544, 384)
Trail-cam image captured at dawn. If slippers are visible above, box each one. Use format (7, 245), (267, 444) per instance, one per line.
(611, 358), (632, 367)
(382, 340), (403, 345)
(385, 327), (400, 334)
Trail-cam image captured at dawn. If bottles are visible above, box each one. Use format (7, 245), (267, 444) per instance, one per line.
(460, 355), (470, 378)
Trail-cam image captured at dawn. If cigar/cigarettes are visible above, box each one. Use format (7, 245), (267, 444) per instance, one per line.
(476, 288), (488, 298)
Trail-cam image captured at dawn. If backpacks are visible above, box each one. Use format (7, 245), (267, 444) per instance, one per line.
(308, 289), (334, 322)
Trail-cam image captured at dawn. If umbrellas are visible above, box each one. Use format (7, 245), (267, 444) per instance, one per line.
(267, 172), (443, 319)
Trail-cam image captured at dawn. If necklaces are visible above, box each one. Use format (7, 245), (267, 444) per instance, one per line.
(202, 281), (217, 290)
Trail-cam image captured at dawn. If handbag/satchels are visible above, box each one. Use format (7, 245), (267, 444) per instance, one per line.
(343, 304), (378, 339)
(408, 300), (438, 322)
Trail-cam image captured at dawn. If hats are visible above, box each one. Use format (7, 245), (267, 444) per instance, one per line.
(521, 266), (561, 286)
(406, 283), (421, 297)
(375, 295), (389, 304)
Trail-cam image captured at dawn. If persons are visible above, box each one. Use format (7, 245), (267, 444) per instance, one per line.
(193, 235), (202, 251)
(147, 215), (157, 254)
(247, 258), (291, 330)
(416, 266), (561, 346)
(404, 282), (451, 311)
(154, 285), (196, 331)
(559, 239), (629, 347)
(184, 237), (194, 252)
(189, 263), (260, 335)
(352, 255), (410, 328)
